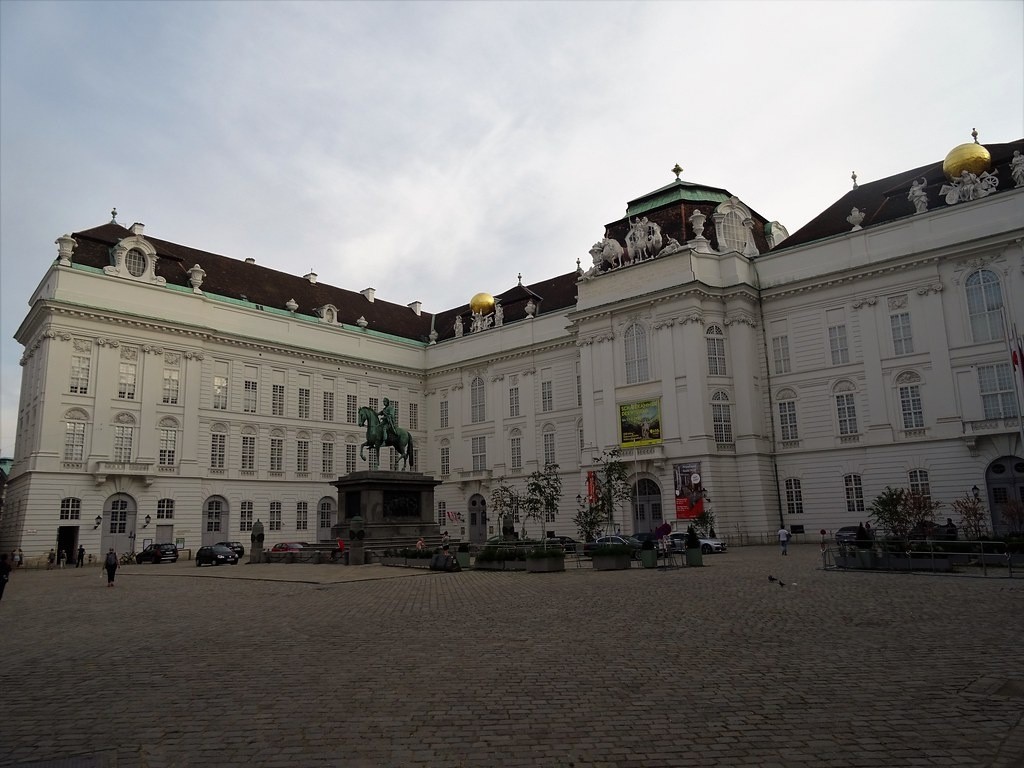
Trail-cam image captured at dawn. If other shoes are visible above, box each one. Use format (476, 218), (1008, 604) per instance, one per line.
(112, 583), (114, 587)
(108, 584), (110, 587)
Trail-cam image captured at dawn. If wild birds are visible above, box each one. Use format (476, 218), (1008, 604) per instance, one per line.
(767, 574), (786, 587)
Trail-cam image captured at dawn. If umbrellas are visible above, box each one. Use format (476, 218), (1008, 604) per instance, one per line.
(657, 524), (671, 539)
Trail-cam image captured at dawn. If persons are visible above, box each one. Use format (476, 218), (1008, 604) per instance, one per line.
(121, 551), (137, 564)
(0, 552), (12, 600)
(376, 397), (398, 445)
(76, 546), (86, 568)
(778, 523), (787, 556)
(854, 522), (867, 540)
(616, 529), (621, 537)
(453, 300), (503, 336)
(576, 527), (605, 540)
(920, 519), (935, 540)
(949, 168), (982, 202)
(10, 547), (23, 568)
(934, 518), (959, 540)
(1009, 150), (1024, 188)
(628, 214), (650, 260)
(102, 548), (121, 587)
(48, 548), (67, 569)
(658, 532), (670, 551)
(907, 177), (928, 213)
(649, 527), (658, 533)
(330, 536), (345, 561)
(441, 531), (449, 554)
(866, 522), (870, 528)
(149, 544), (161, 564)
(416, 534), (426, 551)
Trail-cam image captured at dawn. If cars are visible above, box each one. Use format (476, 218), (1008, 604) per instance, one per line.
(272, 541), (310, 552)
(829, 526), (875, 550)
(195, 544), (239, 566)
(545, 535), (581, 554)
(583, 532), (729, 563)
(215, 541), (244, 558)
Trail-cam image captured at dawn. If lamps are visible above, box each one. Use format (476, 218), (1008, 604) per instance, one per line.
(482, 510), (490, 521)
(94, 515), (102, 529)
(143, 514), (151, 528)
(457, 512), (464, 522)
(701, 488), (710, 503)
(576, 495), (585, 508)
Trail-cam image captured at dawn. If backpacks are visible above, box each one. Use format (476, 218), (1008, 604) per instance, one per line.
(107, 553), (115, 565)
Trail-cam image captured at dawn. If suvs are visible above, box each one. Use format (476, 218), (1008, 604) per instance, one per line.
(136, 543), (179, 564)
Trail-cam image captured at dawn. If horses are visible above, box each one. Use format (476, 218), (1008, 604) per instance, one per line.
(357, 407), (413, 472)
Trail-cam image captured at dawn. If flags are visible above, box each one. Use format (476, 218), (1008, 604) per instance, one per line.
(447, 512), (457, 521)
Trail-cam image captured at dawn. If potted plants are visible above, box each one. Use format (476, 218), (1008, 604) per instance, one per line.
(381, 451), (712, 571)
(835, 520), (1024, 571)
